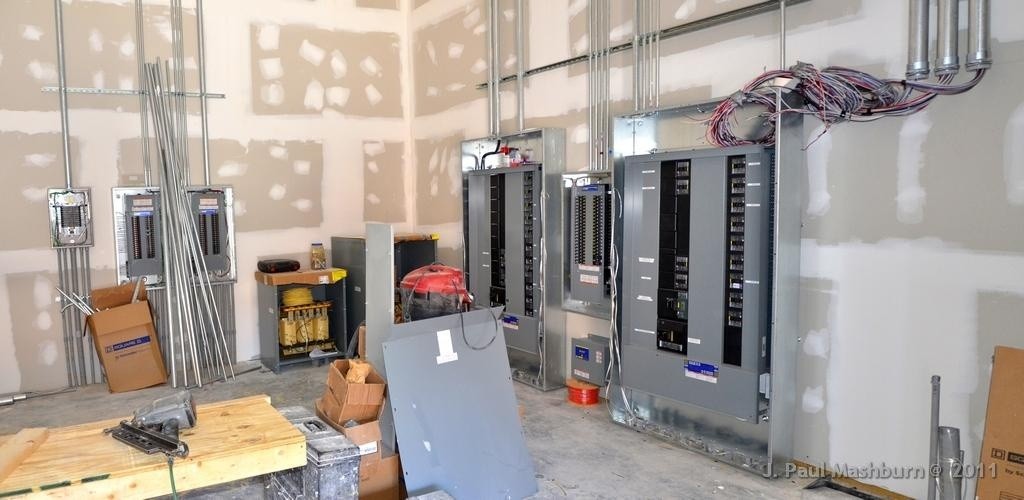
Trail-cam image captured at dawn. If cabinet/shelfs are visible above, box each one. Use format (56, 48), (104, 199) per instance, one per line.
(254, 266), (349, 374)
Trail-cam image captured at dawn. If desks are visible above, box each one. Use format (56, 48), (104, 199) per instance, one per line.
(0, 394), (308, 500)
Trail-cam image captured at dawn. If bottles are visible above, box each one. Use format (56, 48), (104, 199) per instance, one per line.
(310, 242), (327, 269)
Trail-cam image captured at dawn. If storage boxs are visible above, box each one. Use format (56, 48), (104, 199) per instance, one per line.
(311, 355), (402, 499)
(81, 279), (168, 395)
